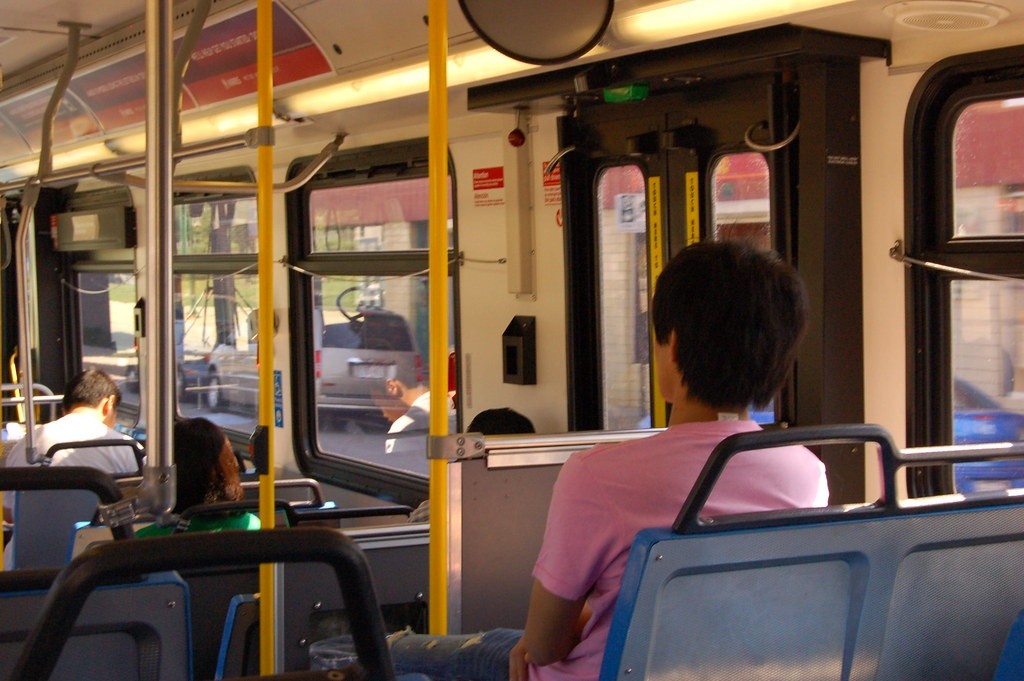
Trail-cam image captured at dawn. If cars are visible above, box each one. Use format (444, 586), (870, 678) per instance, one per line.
(637, 376), (1023, 495)
(125, 319), (231, 402)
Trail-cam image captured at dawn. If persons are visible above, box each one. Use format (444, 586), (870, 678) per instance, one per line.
(369, 357), (457, 454)
(385, 238), (830, 681)
(3, 368), (148, 571)
(135, 417), (262, 539)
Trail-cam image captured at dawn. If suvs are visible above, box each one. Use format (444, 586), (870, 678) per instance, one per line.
(206, 303), (425, 437)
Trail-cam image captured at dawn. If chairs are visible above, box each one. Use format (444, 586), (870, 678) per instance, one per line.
(0, 422), (1024, 681)
